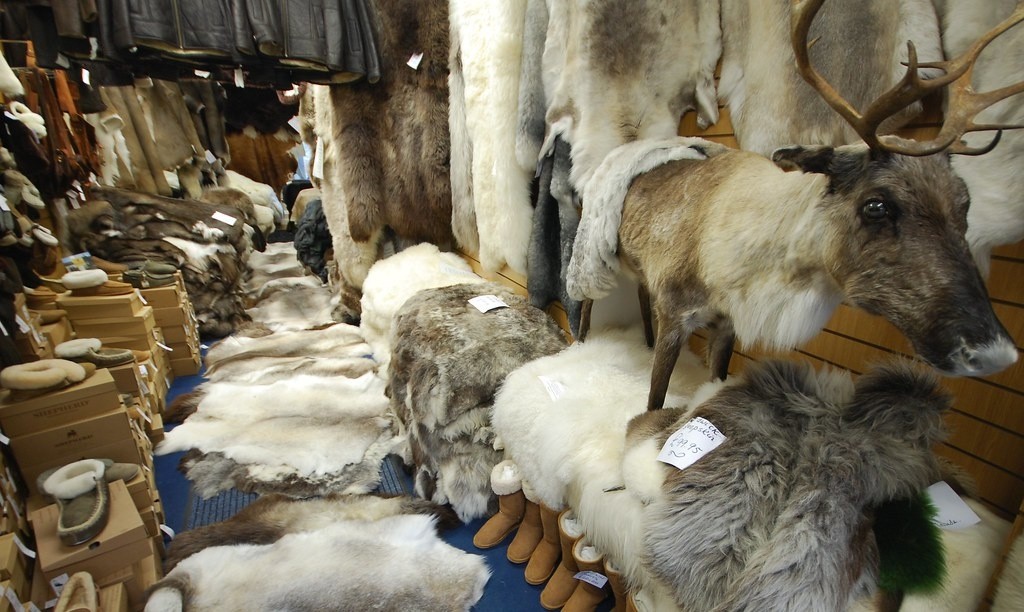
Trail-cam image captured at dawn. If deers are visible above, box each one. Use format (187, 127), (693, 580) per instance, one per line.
(562, 1), (1024, 413)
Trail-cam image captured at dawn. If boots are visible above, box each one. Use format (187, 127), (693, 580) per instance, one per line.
(1, 257), (177, 612)
(472, 459), (655, 612)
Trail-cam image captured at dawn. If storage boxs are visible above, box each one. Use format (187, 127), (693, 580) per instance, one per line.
(0, 266), (203, 612)
